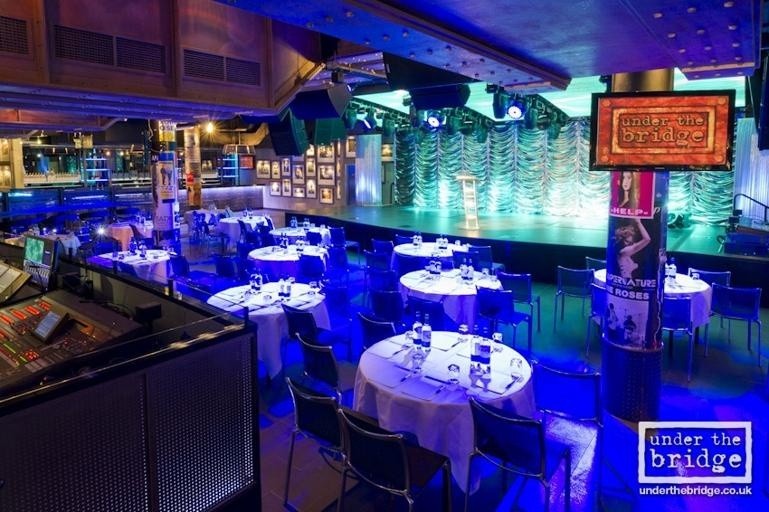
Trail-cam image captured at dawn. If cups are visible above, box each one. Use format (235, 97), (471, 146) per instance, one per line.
(692, 272), (699, 287)
(400, 321), (523, 383)
(307, 281), (318, 301)
(420, 264), (498, 290)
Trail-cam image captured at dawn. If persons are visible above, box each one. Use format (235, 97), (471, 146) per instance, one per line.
(165, 167), (171, 185)
(606, 302), (620, 333)
(622, 314), (636, 341)
(159, 165), (165, 185)
(617, 172), (642, 211)
(611, 217), (650, 285)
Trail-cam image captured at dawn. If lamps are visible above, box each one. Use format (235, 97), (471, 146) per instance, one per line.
(506, 101), (526, 119)
(427, 112), (445, 127)
(358, 116), (376, 132)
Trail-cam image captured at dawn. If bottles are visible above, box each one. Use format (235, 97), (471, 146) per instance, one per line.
(411, 312), (432, 351)
(429, 253), (475, 283)
(86, 148), (110, 189)
(664, 256), (678, 288)
(411, 230), (449, 253)
(469, 325), (491, 377)
(247, 267), (292, 302)
(129, 206), (253, 259)
(276, 215), (311, 252)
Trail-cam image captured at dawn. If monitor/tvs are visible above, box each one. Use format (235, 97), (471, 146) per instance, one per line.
(20, 234), (60, 292)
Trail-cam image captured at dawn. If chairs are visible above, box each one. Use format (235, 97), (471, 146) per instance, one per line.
(711, 283), (763, 367)
(687, 268), (732, 358)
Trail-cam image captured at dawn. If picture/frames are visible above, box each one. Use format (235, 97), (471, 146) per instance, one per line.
(238, 134), (394, 205)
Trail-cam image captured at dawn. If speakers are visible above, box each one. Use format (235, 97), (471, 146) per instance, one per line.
(289, 81), (352, 120)
(409, 84), (472, 110)
